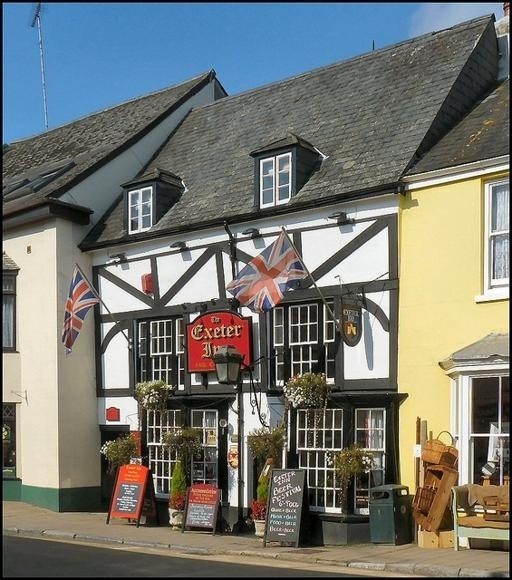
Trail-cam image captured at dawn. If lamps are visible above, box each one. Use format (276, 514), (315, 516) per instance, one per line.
(170, 241), (186, 251)
(328, 210), (346, 220)
(242, 228), (260, 237)
(110, 252), (127, 264)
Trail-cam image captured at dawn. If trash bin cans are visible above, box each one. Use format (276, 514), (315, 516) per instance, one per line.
(369, 484), (411, 546)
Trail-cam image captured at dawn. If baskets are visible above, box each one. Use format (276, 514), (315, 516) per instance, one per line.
(422, 431), (459, 468)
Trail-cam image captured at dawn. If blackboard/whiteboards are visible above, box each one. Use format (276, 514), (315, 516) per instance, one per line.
(264, 469), (305, 543)
(182, 483), (220, 528)
(108, 464), (150, 517)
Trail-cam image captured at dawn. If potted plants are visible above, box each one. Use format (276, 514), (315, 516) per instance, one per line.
(250, 475), (270, 538)
(168, 460), (190, 531)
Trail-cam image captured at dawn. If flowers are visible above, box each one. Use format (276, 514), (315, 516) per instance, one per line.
(98, 431), (139, 467)
(134, 380), (168, 414)
(282, 371), (328, 456)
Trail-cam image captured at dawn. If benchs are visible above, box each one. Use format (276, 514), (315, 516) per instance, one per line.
(450, 483), (509, 553)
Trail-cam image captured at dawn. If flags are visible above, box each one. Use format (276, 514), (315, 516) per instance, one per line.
(225, 226), (311, 319)
(61, 261), (102, 360)
